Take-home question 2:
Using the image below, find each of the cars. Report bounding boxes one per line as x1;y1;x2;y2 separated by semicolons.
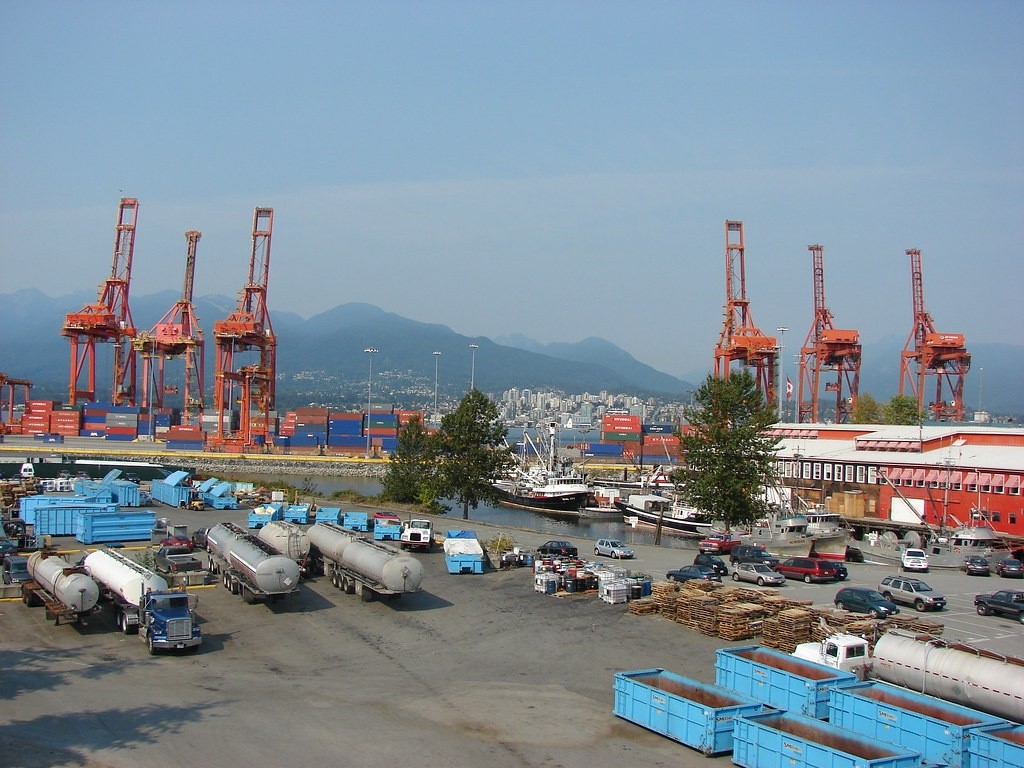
537;540;578;558
773;557;835;583
374;511;400;522
834;587;897;620
666;565;722;584
964;556;990;575
593;539;634;559
994;558;1024;578
160;536;194;554
731;562;786;586
830;561;848;581
694;554;728;576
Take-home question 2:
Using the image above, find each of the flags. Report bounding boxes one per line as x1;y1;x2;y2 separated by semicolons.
785;373;793;400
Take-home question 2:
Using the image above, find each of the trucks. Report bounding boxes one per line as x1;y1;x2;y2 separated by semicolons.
400;519;434;553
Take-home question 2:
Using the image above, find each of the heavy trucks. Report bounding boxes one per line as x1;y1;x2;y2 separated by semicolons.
787;626;1024;725
76;548;203;655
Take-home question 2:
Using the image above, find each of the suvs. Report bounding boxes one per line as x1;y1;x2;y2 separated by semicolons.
2;556;33;585
192;528;211;548
729;545;780;568
900;548;929;572
878;575;946;612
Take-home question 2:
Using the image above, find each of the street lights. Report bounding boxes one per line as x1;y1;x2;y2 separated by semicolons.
433;351;441;427
363;348;379;459
469;342;479;391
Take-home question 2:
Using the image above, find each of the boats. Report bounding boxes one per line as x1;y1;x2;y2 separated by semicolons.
480;421;1024;572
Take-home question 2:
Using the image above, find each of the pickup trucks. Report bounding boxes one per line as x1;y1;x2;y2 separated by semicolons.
973;590;1024;624
153;546;203;574
698;533;741;555
